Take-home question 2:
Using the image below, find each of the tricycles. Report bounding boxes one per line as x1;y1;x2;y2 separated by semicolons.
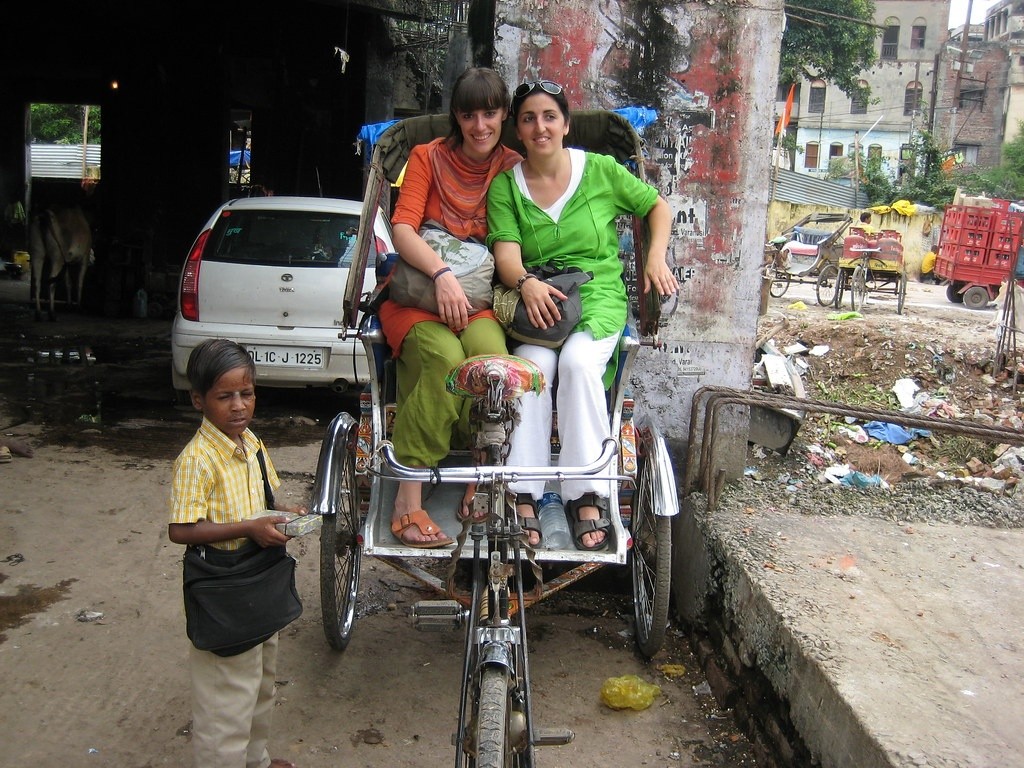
763;211;908;312
310;106;685;768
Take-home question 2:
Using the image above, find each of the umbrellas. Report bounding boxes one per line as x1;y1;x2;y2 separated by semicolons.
230;150;250;167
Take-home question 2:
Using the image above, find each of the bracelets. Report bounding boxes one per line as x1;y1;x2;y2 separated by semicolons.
432;267;452;283
515;273;537;293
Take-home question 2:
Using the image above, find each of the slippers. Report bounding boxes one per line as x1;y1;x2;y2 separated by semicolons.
391;509;454;549
457;490;488;524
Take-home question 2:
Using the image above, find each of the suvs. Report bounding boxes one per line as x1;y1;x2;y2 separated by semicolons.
170;195;399;415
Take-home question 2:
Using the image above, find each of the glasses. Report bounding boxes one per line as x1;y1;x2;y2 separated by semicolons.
513;80;566;98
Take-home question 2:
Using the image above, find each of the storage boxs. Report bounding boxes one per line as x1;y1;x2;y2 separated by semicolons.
940;185;1024;268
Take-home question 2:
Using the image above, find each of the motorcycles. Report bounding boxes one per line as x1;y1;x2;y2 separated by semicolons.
932;251;1024;310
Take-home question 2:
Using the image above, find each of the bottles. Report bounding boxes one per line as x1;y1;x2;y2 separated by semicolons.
537;491;570;550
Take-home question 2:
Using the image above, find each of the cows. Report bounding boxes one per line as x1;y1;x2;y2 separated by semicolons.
28;200;92;322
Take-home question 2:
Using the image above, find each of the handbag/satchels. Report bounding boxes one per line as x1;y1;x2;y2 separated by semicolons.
182;535;304;657
390;217;495;318
493;257;592;348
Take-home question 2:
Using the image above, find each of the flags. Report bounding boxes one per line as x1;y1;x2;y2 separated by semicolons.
774;84;796;135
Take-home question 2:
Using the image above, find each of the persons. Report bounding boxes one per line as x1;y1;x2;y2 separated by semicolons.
377;67;524;549
486;80;680;552
858;211;876;235
168;339;310;768
920;245;941;285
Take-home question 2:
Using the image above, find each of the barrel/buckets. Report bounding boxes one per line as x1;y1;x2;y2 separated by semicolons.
14;251;30;273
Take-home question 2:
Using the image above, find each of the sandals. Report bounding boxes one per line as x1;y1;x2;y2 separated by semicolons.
510;493;543;549
566;494;610;551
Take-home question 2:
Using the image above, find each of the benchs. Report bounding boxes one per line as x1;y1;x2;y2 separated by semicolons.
368;255;639;404
844;227;901;262
785;226;833;255
